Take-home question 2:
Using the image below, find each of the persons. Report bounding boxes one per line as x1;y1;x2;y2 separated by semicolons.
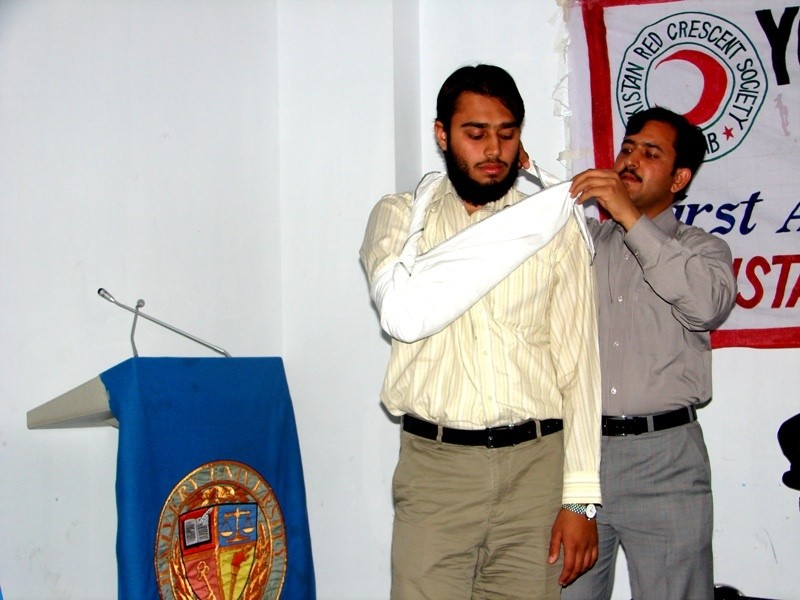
520;106;735;600
360;66;602;600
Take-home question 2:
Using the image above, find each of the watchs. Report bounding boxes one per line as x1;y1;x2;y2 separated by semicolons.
562;503;597;520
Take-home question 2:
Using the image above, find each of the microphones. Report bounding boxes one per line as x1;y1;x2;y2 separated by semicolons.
98;288;232;358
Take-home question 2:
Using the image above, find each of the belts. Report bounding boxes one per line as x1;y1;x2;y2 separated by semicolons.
403;414;563;448
602;404;697;436
